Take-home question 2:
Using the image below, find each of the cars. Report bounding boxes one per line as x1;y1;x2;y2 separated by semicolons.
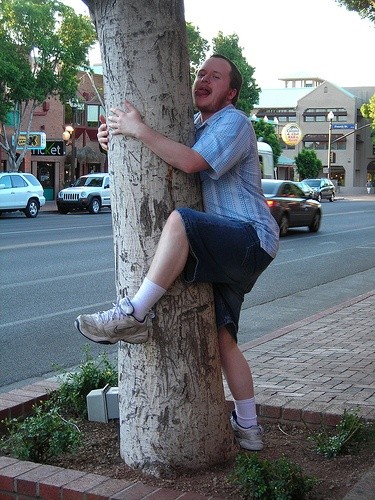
261;178;322;236
301;179;336;203
0;172;46;218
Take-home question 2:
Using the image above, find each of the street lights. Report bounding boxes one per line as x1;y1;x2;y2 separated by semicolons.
62;98;78;184
328;111;334;179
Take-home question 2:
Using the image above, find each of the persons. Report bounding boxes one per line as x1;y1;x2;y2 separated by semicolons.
75;53;280;449
329;174;371;194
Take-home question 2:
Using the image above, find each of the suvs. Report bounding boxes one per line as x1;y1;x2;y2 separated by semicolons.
56;173;111;214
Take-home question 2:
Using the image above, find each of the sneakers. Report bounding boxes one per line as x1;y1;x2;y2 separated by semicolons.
75;297;155;344
230;410;263;450
